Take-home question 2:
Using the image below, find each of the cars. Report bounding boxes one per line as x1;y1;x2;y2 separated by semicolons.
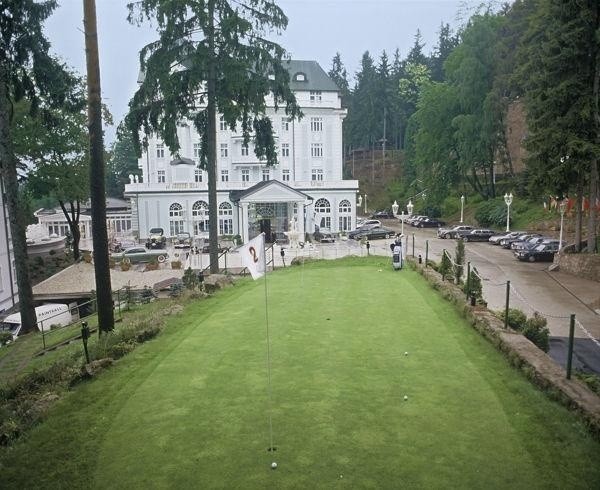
146;228;166;248
348;219;395;240
173;232;191;248
406;215;446;228
489;231;567;263
370;211;395;219
437;225;495;242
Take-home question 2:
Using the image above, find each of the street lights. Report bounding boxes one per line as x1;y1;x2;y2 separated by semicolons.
559;201;567;251
460;195;464;222
392;200;414;234
504;192;513;232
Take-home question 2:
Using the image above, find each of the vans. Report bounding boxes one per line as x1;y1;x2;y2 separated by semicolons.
0;304;71;344
